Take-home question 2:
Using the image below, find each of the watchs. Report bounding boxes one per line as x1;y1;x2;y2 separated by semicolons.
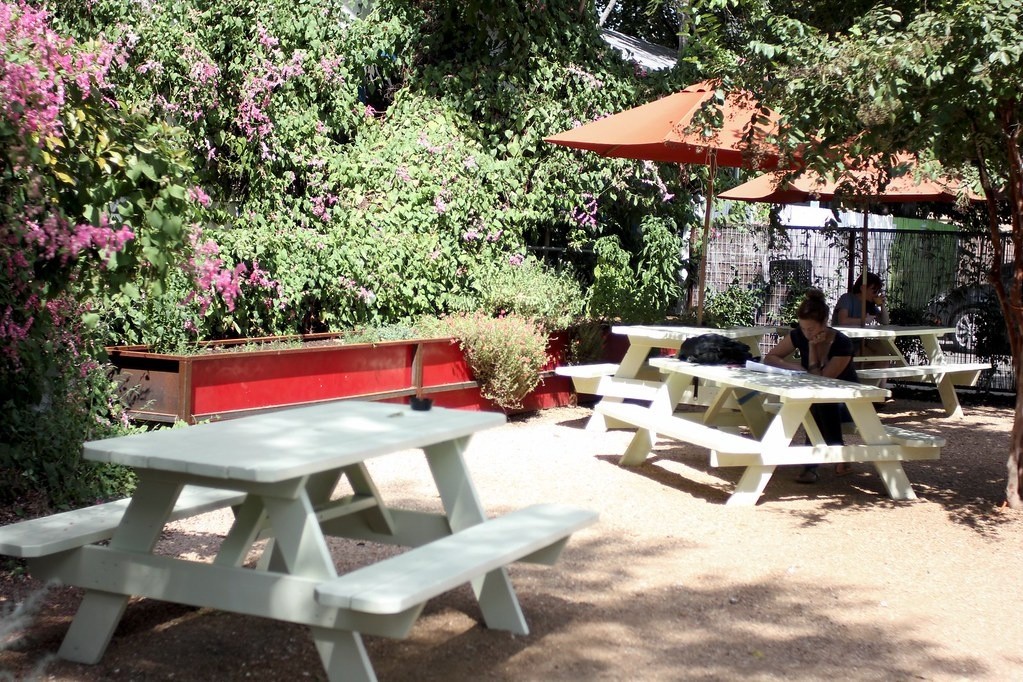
808;360;821;371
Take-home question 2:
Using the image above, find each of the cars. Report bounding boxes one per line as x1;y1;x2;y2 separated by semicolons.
922;284;999;354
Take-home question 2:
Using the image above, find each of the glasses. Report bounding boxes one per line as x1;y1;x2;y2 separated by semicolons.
869;287;881;294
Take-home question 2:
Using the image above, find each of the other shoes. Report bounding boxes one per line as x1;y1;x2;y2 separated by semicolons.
831;463;855;477
794;468;823;483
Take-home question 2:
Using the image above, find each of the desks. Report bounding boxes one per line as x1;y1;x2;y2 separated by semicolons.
55;400;530;682
619;358;918;509
585;326;779;438
777;325;982;417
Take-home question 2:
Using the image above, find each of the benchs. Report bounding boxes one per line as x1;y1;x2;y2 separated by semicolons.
852;356;901;363
763;404;945;448
556;363;621;379
314;503;599;613
856;362;992;381
595;402;762;453
0;483;248;558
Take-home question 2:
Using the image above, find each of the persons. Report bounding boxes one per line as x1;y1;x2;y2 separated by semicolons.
763;290;860;484
831;272;895;411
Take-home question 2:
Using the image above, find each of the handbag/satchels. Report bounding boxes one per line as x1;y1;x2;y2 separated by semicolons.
675;332;756;366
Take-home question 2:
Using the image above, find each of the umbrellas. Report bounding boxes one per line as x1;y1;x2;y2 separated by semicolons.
717;140;988;328
544;77;874;327
